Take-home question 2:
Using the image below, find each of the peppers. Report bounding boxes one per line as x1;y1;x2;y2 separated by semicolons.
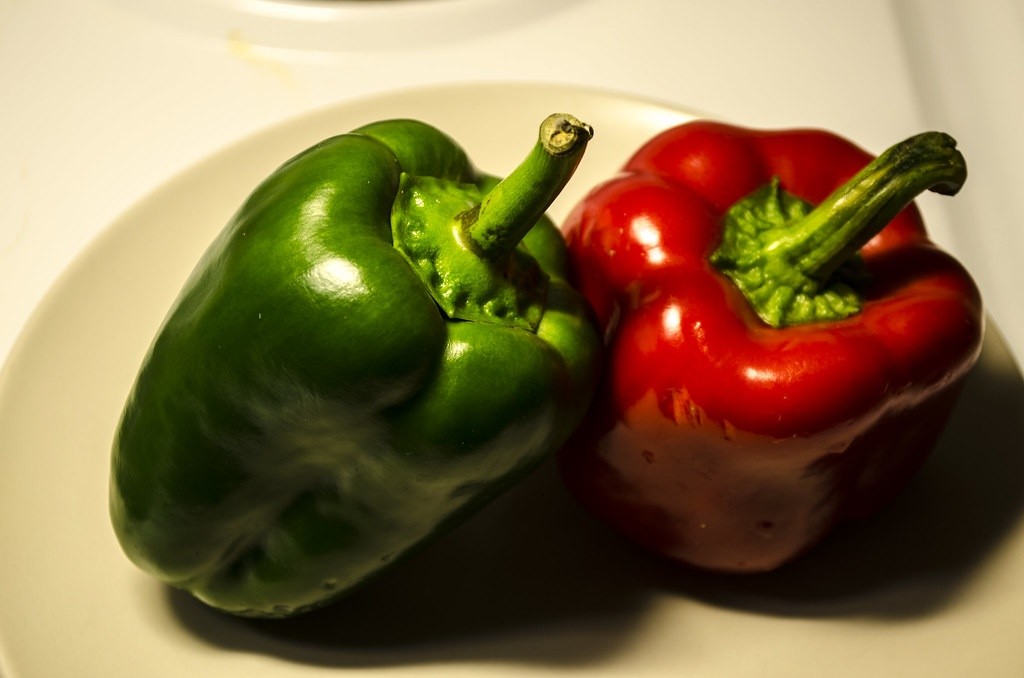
554;117;985;574
107;112;599;619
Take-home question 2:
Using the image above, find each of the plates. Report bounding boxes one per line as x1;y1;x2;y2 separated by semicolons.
0;81;1024;678
